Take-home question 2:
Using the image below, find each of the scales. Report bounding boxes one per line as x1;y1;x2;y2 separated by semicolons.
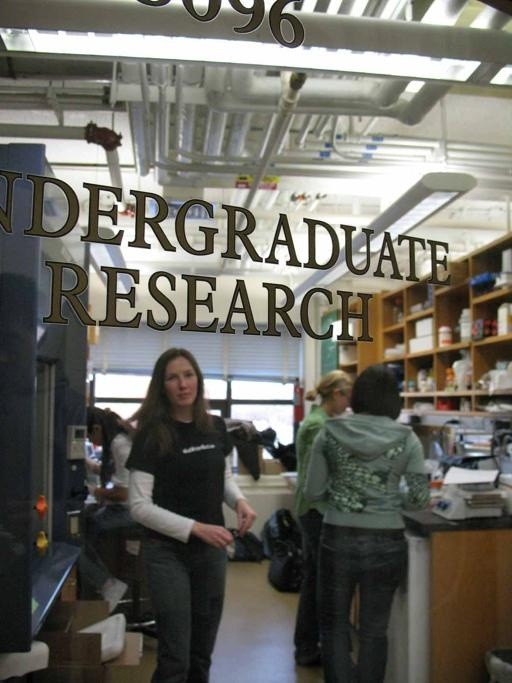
432;488;505;520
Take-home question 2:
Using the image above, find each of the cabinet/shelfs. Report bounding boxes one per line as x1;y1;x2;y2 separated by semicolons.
338;230;512;418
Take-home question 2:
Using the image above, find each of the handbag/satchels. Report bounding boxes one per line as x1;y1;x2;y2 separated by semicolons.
227;529;264;561
261;510;302;561
268;540;305;592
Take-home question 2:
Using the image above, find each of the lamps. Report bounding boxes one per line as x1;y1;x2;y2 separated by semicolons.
281;98;479;308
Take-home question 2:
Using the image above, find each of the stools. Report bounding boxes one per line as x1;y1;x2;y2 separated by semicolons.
80;503;158;632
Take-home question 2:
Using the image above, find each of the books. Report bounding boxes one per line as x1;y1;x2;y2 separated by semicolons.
443;466;507;509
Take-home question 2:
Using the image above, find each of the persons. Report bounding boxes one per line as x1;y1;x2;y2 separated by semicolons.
124;347;258;683
293;369;355;667
79;406;139;616
303;365;430;683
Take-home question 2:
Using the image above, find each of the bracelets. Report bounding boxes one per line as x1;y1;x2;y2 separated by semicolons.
93;463;97;474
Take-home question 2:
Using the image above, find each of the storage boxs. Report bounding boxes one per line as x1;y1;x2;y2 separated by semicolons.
34;600;160;683
59;565;77;604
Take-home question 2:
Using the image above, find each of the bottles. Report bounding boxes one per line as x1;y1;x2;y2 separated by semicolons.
438;325;452;346
417;367;427;392
457;309;471;344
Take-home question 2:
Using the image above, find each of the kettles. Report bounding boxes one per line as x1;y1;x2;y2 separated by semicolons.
441;419;465;458
